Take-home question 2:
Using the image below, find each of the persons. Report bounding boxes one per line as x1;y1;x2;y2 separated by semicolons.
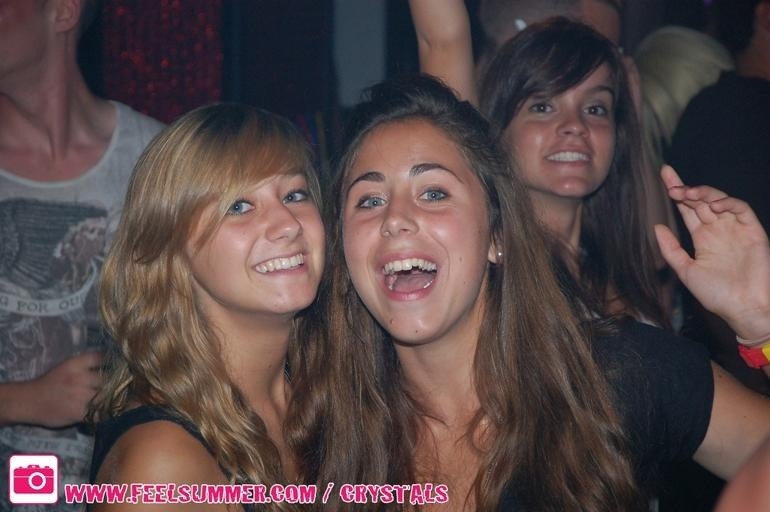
274;70;770;511
472;15;700;347
0;1;174;512
87;98;332;511
408;0;643;124
660;0;770;396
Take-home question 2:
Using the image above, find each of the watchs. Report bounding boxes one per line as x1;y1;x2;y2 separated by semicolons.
736;341;770;371
